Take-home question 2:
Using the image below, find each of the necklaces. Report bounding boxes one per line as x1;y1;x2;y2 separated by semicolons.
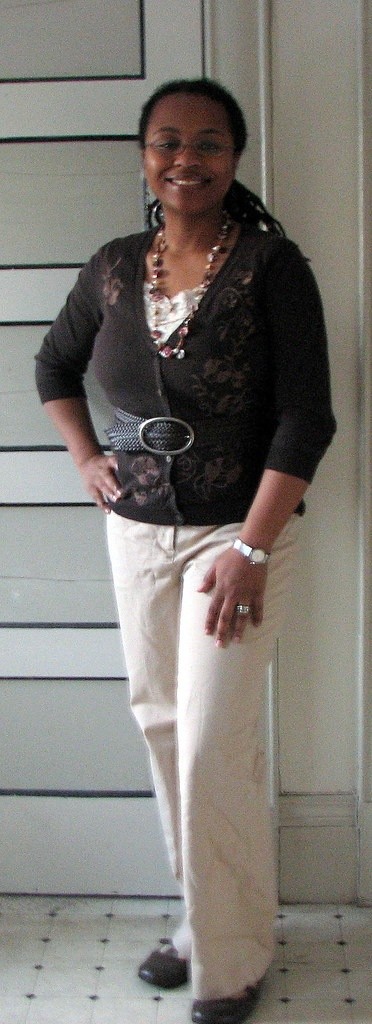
149;219;233;359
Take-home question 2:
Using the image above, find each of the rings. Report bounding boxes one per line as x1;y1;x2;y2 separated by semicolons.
237;605;251;613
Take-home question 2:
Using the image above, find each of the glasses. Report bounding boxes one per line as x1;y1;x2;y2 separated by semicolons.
143;137;237;158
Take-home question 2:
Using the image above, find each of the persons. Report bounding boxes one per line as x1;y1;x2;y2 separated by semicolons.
34;76;337;1024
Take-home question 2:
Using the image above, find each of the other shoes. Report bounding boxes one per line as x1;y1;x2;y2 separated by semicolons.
136;937;191;990
190;973;266;1024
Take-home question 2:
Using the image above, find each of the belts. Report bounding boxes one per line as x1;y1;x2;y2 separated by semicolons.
102;405;258;456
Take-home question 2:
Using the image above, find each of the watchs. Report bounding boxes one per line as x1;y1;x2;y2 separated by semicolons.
233;537;270;565
